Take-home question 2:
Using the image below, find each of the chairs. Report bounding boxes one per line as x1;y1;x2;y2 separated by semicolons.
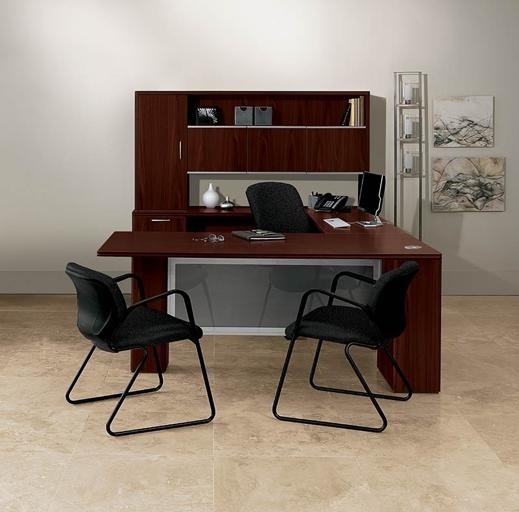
65;262;216;436
258;266;333;326
246;182;308;234
321;269;364;300
271;262;418;434
175;264;215;327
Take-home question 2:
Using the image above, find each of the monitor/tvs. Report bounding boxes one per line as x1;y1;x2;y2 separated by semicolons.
357;170;386;225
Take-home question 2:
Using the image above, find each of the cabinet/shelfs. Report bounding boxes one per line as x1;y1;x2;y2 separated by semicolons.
395;71;424;243
135;91;370;229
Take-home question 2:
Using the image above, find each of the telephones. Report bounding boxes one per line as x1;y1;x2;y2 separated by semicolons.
314;193;348;211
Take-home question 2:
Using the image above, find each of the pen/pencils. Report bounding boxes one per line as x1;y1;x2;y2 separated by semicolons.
311;191;318;196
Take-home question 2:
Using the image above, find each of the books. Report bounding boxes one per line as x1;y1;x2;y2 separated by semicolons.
231;229;287;241
338;94;364;128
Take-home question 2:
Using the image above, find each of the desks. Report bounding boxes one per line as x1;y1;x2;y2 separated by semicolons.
97;208;442;393
